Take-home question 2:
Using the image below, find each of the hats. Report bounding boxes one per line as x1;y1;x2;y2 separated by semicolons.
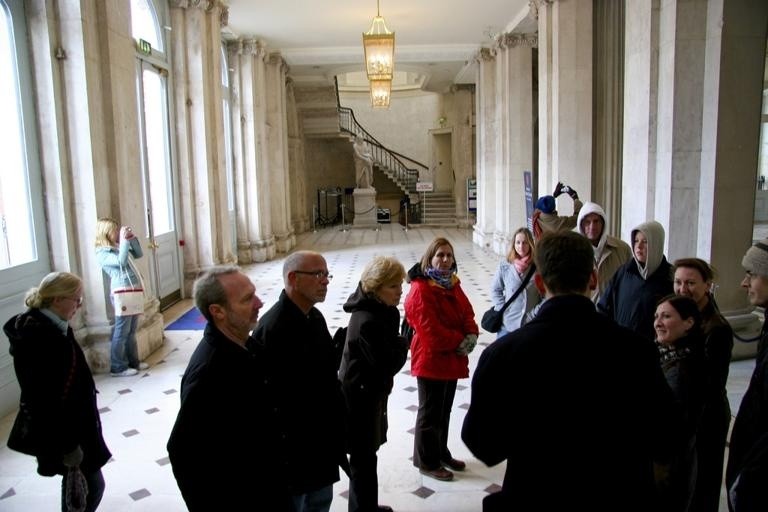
64;468;91;512
739;233;768;277
536;196;556;214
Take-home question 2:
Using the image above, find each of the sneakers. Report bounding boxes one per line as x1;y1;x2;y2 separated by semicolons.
137;360;150;370
109;367;139;377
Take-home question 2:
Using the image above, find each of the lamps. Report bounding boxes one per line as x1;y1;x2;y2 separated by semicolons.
369;80;393;110
357;0;395;80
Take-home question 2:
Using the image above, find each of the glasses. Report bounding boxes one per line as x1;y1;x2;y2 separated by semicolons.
292;270;334;280
56;295;84;305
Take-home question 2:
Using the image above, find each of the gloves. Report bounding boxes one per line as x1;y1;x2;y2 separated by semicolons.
567;186;578;199
553;182;565;197
62;447;86;469
455;334;476;357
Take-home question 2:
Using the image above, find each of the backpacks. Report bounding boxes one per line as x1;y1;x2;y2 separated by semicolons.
400;311;415;348
332;326;349;371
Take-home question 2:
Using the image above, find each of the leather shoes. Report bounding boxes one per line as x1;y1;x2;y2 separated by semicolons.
418;466;455;482
441;458;465;472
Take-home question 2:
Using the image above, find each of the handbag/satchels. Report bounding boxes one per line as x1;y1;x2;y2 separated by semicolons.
480;306;505;334
112;287;145;317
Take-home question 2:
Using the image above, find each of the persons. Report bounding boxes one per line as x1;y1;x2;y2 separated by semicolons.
725;238;768;511
353;132;375;189
3;271;112;511
252;251;345;511
653;295;713;511
577;201;632;312
339;255;413;510
534;181;584;232
492;227;548;340
95;216;150;375
461;230;695;511
599;219;676;345
673;258;734;511
405;237;478;481
166;264;309;510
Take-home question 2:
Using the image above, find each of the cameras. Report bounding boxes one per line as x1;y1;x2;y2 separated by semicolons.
125;226;134;233
561;186;570;193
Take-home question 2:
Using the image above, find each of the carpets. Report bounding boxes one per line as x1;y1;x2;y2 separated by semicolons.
166;299;211;333
